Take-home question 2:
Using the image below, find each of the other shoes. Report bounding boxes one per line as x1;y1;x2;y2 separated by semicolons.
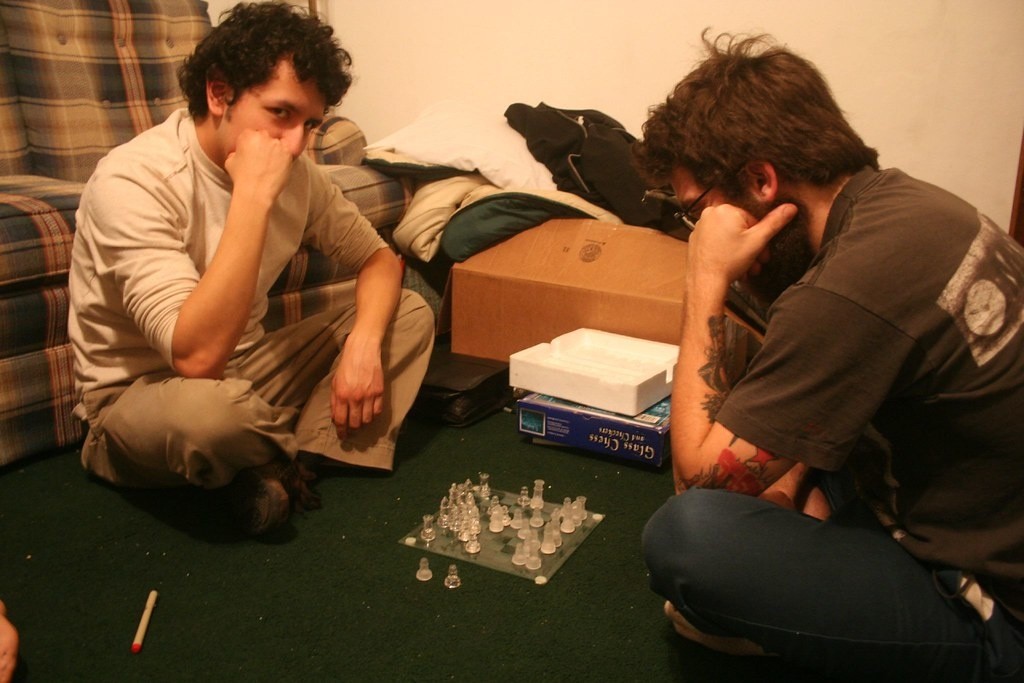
662;600;777;656
231;458;321;533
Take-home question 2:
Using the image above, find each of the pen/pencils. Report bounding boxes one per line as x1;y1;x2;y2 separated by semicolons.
131;586;161;651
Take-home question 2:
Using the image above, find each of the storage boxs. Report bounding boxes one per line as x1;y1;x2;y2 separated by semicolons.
516;393;672;470
435;218;689;362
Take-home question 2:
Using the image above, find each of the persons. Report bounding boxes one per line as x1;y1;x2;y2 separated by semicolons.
633;47;1024;683
67;0;437;534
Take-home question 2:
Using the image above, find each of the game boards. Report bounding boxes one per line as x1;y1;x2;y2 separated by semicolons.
396;485;608;585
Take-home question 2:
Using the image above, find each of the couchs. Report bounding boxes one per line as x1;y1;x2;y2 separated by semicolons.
0;0;435;465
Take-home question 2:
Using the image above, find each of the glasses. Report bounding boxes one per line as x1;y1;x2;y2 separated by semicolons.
673;181;720;231
